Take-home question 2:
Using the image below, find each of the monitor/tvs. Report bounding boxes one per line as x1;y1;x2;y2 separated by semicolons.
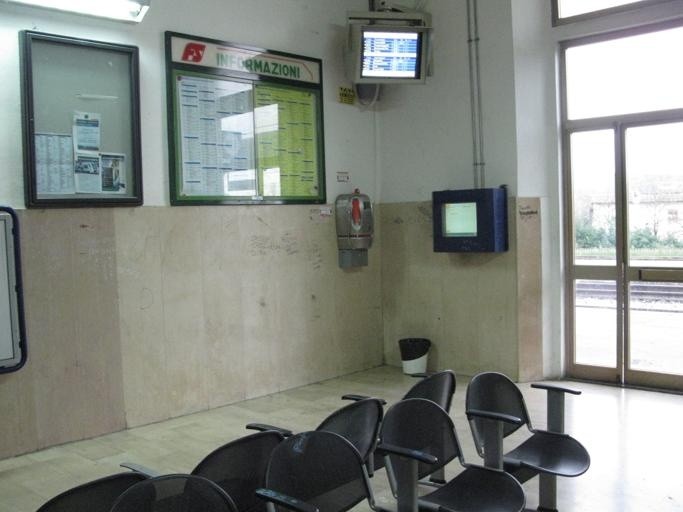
346;23;428;84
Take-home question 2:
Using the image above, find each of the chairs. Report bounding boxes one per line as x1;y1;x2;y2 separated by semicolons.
255;427;439;512
247;395;387;501
343;369;459;498
112;473;318;512
39;461;170;512
380;399;528;511
120;421;295;512
467;371;590;511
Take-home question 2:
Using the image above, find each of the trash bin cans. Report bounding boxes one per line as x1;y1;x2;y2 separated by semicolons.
399;338;431;374
